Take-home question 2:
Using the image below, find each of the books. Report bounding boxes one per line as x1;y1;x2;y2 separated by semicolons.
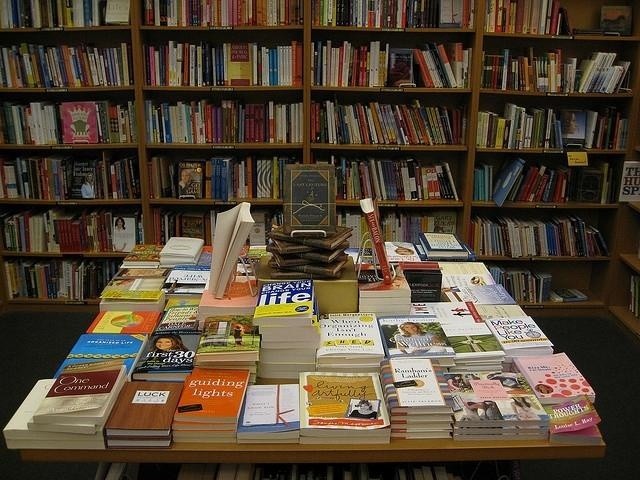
144;1;304;243
0;197;601;478
310;1;475;247
281;164;338;238
0;1;144;298
468;2;638;317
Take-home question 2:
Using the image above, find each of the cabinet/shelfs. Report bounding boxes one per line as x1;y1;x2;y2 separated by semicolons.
1;0;639;340
